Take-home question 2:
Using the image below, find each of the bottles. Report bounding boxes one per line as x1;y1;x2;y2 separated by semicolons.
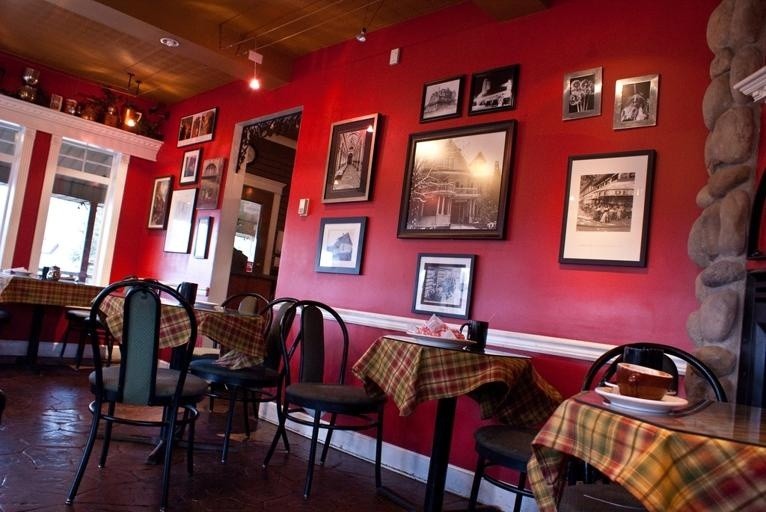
46;265;61;281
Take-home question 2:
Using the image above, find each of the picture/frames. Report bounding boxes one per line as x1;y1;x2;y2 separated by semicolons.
558;148;655;267
148;175;174;230
410;252;477;321
180;147;202;184
163;189;198;254
314;216;368;275
563;66;603;120
397;118;517;240
196;156;225;210
467;63;521;116
419;73;464;124
194;215;211;259
612;72;660;130
320;112;381;204
177;108;217;148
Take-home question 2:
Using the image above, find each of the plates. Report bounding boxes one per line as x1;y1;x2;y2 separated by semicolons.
194;301;218;310
407;332;477;352
595;387;688;414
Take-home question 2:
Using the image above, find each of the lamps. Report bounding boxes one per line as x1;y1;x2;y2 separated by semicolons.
354;33;366;43
161;37;180;48
124;106;142;127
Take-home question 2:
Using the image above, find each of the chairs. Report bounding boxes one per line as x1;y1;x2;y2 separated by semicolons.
64;278;209;512
59;277;157;368
574;341;727;512
259;300;385;501
169;292;273;428
472;341;629;512
188;296;304;465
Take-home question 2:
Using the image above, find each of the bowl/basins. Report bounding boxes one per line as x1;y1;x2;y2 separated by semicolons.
616;362;672;401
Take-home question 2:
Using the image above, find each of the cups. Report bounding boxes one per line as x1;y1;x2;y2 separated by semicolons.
175;281;198;308
459;320;489;352
622;347;665;374
